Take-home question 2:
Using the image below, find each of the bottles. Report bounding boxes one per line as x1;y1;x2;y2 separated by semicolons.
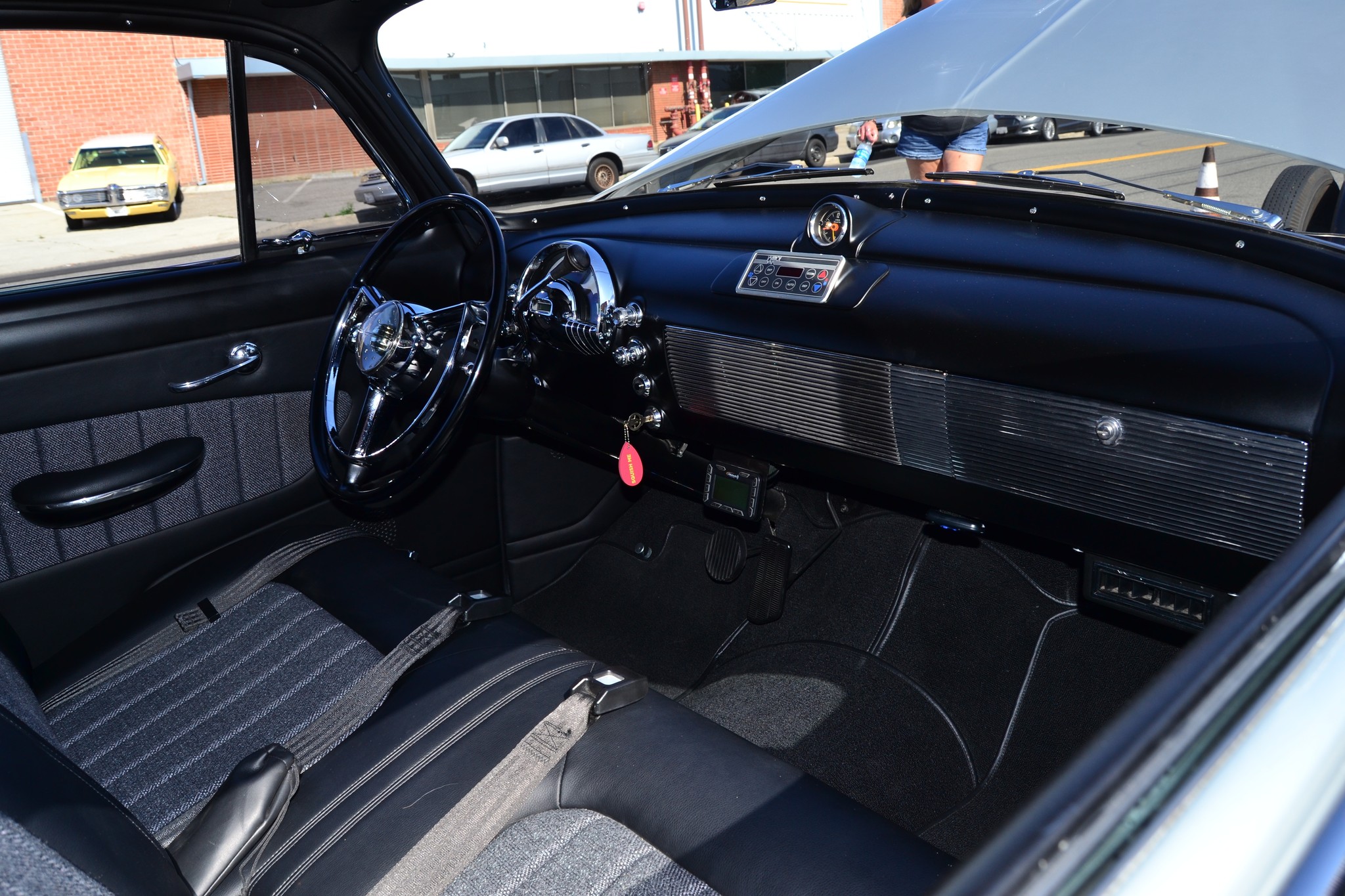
849;139;872;178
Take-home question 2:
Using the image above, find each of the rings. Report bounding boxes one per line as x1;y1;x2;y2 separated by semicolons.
865;129;870;132
872;134;878;137
857;134;861;137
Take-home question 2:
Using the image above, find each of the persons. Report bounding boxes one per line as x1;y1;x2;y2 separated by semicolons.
856;0;997;185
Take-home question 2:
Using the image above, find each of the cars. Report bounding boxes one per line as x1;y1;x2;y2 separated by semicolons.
993;115;1143;142
657;101;838;177
354;112;659;215
847;114;999;157
1;0;1345;895
57;133;184;230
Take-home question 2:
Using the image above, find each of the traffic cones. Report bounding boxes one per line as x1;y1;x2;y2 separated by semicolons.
1189;146;1222;217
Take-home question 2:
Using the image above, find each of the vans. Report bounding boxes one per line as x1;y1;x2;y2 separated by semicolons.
730;87;775;105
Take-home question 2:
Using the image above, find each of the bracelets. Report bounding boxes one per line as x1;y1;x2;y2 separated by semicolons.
860;119;876;126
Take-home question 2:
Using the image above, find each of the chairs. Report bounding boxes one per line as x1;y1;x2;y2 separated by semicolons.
0;493;970;896
